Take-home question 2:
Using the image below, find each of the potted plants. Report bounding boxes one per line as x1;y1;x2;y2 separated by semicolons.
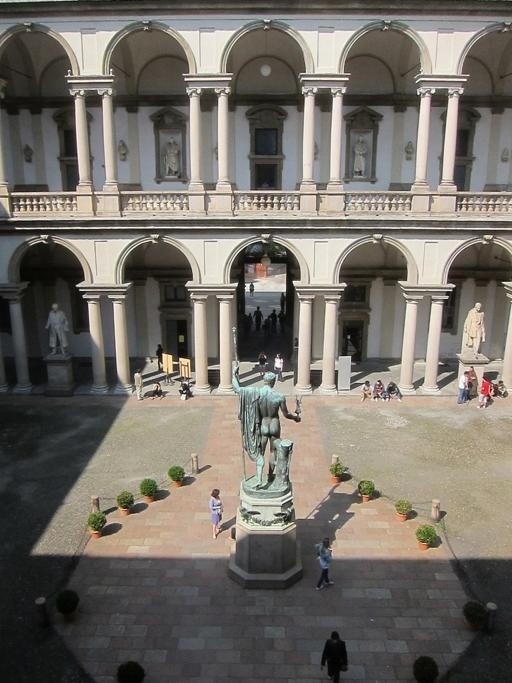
168;466;186;486
328;463;349;484
394;499;412;521
116;491;134;515
87;511;107;539
358;480;375;502
463;600;488;631
415;524;437;549
54;590;80;617
140;478;157;503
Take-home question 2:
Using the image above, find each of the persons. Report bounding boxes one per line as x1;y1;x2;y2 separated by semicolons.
178;384;193;398
258;351;267;380
386;382;401;401
243;306;285;336
164;137;180;176
45;303;69;356
464;303;485;359
209;489;224;539
232;361;301;490
280;292;286;312
315;538;334;590
352;135;368;176
152;382;163;398
321;631;348;683
457;365;506;408
250;282;254;297
373;380;386;402
156;344;163;371
360;381;375;402
134;369;143;400
274;354;284;383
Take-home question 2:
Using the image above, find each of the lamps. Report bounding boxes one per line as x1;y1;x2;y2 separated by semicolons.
260;29;272;76
